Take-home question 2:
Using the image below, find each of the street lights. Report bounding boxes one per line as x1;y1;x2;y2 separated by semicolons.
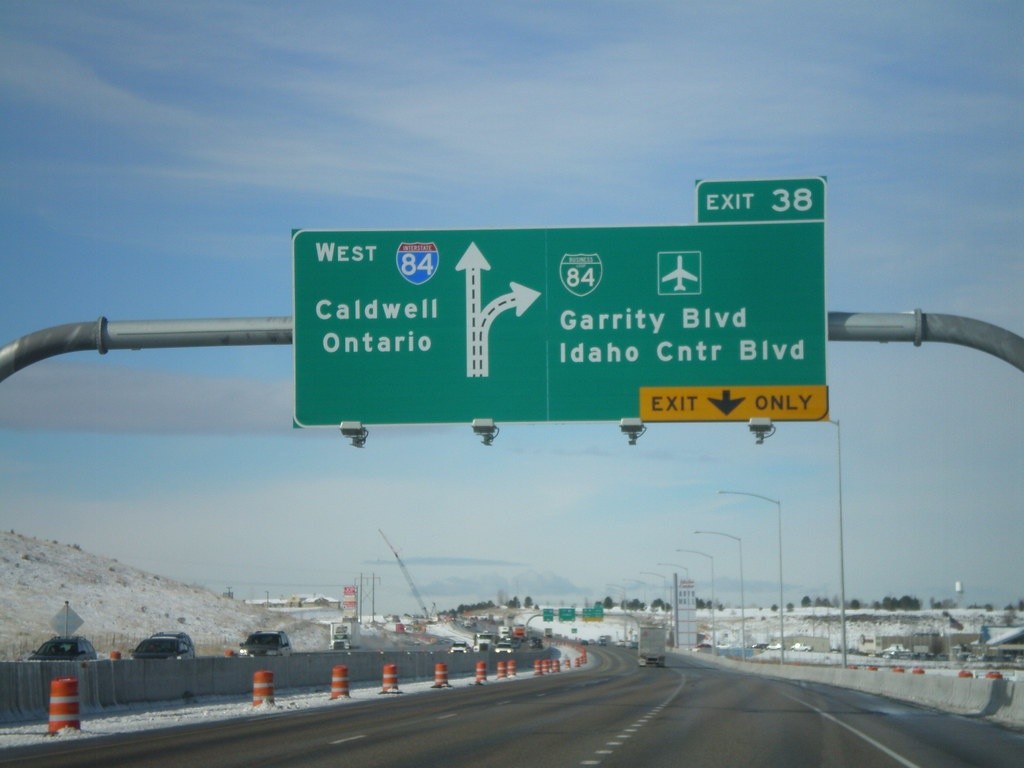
674;488;785;663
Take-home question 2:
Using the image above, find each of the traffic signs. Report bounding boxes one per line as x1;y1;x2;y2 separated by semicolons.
291;220;829;426
543;608;554;621
558;608;576;621
583;608;605;621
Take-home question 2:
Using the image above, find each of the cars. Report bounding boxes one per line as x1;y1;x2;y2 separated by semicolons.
494;642;516;653
863;647;1018;663
691;643;712;653
752;640;815;652
448;641;474;654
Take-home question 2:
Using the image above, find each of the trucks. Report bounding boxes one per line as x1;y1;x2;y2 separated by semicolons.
473;631;494;648
497;624;526;640
598;636;607;647
329;622;362;651
637;624;666;667
544;628;553;638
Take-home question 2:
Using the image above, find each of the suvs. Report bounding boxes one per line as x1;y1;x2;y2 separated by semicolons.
27;635;97;663
237;630;292;658
128;631;197;661
